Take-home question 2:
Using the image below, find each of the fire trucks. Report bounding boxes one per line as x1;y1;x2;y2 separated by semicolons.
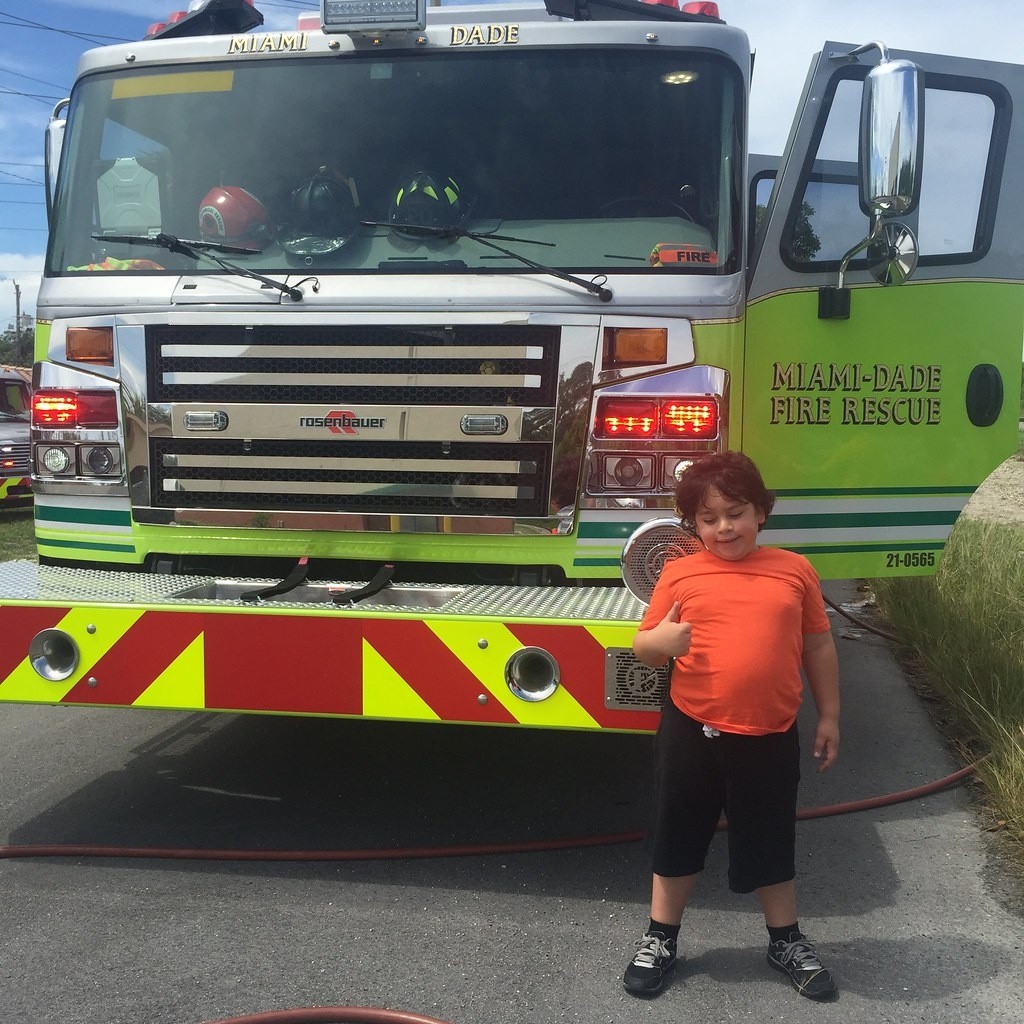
0;0;1024;735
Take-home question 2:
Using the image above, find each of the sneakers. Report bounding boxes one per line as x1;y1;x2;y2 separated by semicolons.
765;930;838;1002
621;930;678;998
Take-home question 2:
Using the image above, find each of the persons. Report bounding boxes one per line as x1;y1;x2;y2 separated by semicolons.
622;452;839;999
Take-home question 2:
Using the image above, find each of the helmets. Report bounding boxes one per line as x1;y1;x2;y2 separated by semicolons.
273;174;360;257
197;186;272;252
390;169;473;242
